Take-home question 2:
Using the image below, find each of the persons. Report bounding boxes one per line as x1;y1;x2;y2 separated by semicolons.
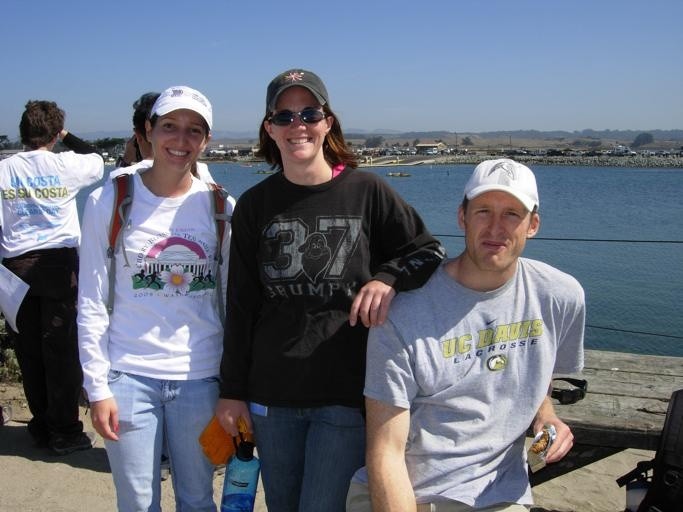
75;86;236;512
344;159;586;512
215;69;447;512
0;101;104;458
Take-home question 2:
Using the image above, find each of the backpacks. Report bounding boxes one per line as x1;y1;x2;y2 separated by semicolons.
615;389;682;512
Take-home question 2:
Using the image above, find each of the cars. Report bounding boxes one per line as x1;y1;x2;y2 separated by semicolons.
379;143;417;155
514;149;527;154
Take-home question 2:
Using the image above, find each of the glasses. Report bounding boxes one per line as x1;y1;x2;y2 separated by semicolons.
551;376;588;406
271;107;325;126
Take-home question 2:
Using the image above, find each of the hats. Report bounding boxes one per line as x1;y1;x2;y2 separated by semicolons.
463;158;539;213
266;69;329;112
149;84;214;144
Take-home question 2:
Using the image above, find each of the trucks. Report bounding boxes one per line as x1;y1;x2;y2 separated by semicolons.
567;146;683;157
426;144;468;155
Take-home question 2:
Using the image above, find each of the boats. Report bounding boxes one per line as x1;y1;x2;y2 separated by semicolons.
248;171;273;175
385;172;411;177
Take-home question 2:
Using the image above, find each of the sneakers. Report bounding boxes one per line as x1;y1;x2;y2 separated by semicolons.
49;430;98;456
2;406;12;422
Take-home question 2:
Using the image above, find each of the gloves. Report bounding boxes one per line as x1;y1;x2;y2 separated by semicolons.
199;413;251;465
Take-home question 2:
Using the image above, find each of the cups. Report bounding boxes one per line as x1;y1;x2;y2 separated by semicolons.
220;430;260;512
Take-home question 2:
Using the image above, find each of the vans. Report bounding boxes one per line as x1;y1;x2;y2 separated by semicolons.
546;148;565;156
